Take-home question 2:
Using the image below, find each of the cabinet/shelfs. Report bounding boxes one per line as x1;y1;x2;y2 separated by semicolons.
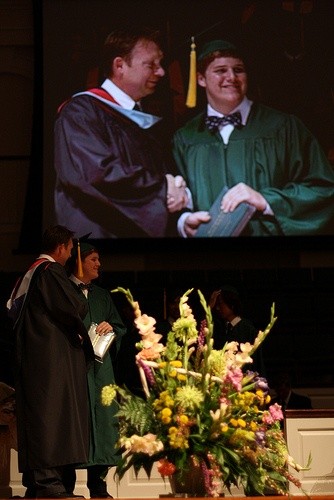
8;409;334;499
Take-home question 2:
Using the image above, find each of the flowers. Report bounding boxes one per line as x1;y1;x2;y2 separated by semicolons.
96;286;314;500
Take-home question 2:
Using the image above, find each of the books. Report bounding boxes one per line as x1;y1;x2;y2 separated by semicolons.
192;182;257;233
82;321;116;364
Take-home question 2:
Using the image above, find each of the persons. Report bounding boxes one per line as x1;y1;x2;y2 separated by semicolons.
151;287;271;416
7;225;126;499
53;27;333;235
